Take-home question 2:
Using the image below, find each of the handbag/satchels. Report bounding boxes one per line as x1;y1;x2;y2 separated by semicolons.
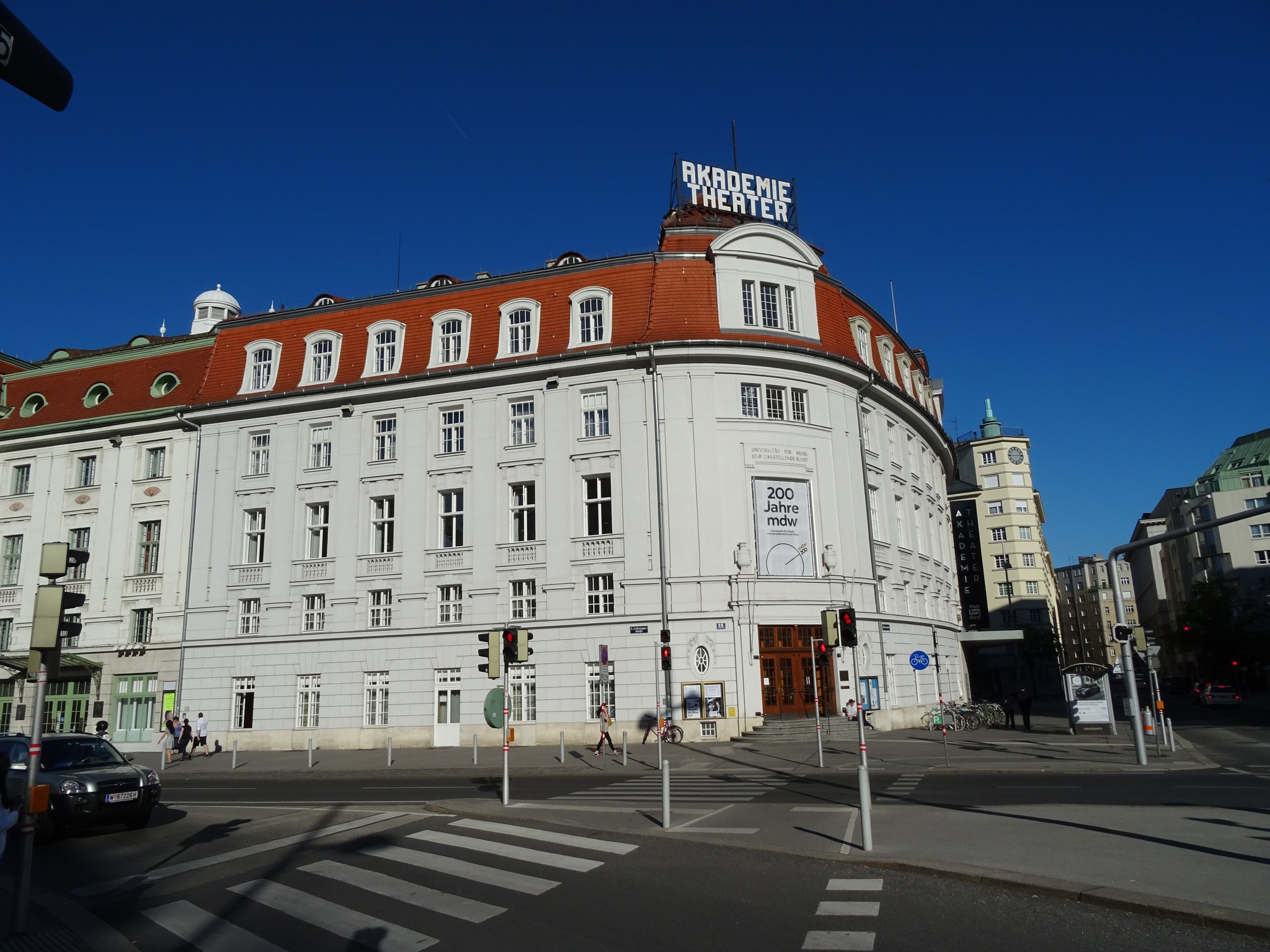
189;735;193;742
608;717;612;726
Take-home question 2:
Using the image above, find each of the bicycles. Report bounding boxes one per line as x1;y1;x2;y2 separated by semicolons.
642;714;684;745
921;697;1006;731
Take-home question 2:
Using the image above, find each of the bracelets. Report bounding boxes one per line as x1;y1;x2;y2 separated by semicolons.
197;736;200;738
178;739;180;741
159;740;160;742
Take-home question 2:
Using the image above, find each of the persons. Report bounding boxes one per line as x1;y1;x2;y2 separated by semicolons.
0;750;24;860
94;720;112;759
1001;686;1034;729
158;712;209;765
708;701;722;718
595;702;621;755
846;699;874;729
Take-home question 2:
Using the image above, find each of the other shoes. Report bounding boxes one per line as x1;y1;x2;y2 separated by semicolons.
872;727;879;730
594;753;602;756
173;752;178;754
179;758;185;761
165;761;172;764
203;754;210;759
614;751;621;756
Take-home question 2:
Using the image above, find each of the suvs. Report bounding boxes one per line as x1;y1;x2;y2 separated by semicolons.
1075;682;1100;698
0;730;162;843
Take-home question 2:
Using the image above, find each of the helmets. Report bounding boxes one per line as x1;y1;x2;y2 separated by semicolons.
96;721;108;732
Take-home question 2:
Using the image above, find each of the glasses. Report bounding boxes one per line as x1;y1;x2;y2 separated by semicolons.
605;706;608;708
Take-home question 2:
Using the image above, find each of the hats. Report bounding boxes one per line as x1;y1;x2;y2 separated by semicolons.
166;720;173;726
849;699;855;703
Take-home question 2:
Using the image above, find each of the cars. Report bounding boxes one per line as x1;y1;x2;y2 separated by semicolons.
1134;673;1195;695
1196;684;1240;709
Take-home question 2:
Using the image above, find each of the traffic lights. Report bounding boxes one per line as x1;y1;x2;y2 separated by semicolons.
838;608;858;648
1182;622;1189;634
30;585;86;651
478;629;500;680
517;629;533;664
661;646;671;670
39;541;90;580
502;627;518;663
1112;623;1133;643
817;639;831;667
